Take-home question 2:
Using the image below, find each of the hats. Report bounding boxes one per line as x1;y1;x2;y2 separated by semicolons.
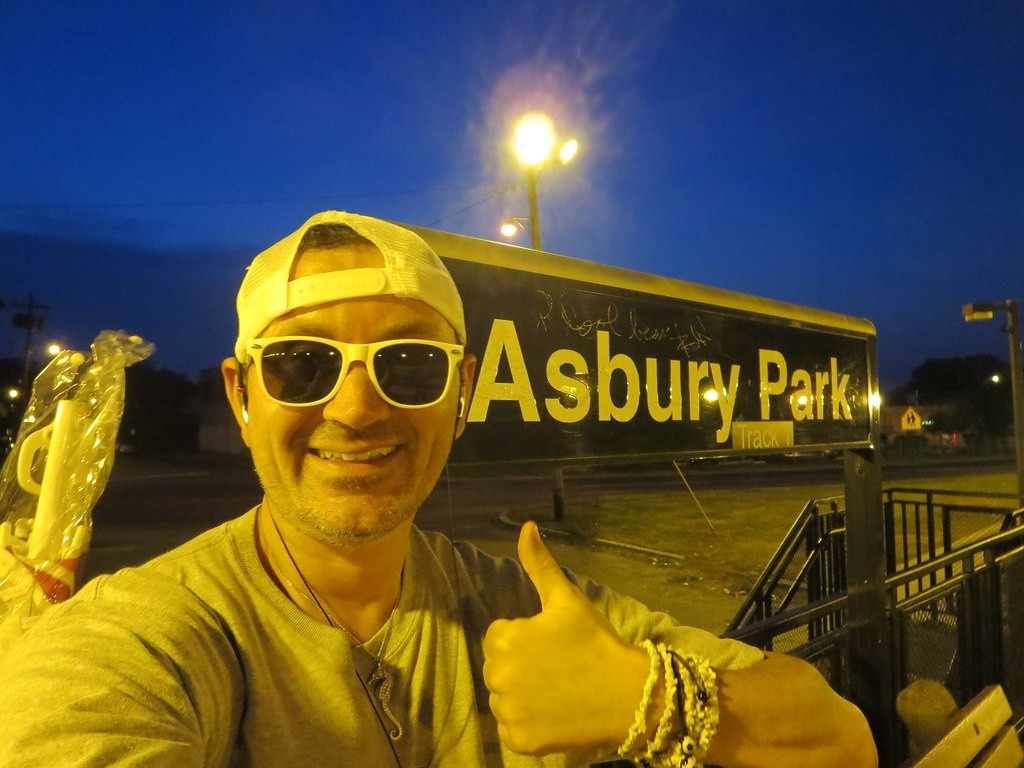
234;210;467;370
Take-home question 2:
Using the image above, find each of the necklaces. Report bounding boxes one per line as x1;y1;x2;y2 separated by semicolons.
287;576;402;740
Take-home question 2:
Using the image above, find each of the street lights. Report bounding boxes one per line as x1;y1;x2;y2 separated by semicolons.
960;297;1023;513
506;113;580;525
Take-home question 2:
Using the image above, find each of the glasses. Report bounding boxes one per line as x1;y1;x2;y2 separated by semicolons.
245;335;464;409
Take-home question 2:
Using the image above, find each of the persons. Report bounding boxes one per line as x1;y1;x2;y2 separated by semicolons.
0;207;882;768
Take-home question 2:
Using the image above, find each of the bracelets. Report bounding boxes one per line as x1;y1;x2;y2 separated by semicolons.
617;637;720;768
